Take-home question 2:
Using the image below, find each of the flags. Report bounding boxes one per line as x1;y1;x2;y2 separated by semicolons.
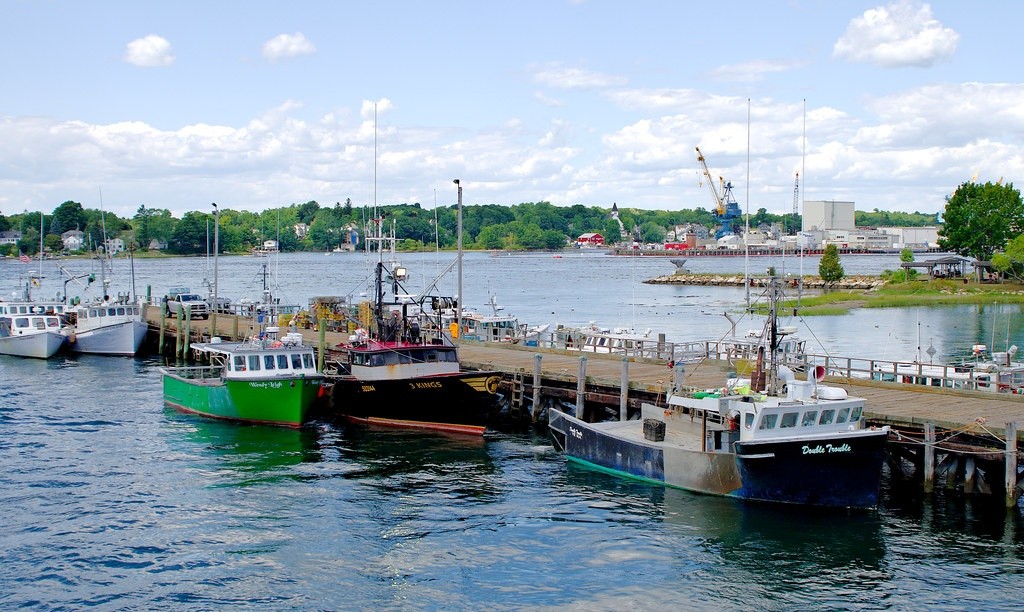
20;252;31;262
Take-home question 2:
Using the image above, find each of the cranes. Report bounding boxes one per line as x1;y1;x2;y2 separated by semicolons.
791;171;800;236
695;145;745;242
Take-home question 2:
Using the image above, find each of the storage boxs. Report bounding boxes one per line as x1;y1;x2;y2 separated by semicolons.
643;419;666;442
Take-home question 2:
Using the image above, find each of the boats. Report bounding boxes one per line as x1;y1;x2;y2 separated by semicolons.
548;97;893;509
0;101;653;440
718;327;808;373
870;299;1024;395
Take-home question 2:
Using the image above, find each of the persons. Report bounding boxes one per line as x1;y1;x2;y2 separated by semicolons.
162;295;170;303
989;272;997;284
311;304;318;331
